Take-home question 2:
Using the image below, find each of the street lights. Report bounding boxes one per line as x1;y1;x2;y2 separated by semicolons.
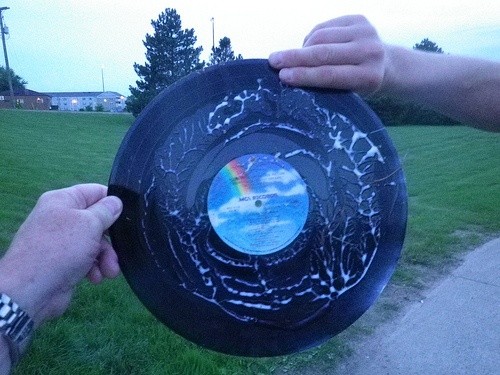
210;16;215;51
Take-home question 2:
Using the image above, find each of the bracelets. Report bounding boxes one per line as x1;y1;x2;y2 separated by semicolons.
0;291;35;365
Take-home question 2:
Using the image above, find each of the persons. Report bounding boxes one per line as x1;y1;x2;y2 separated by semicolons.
0;15;500;375
